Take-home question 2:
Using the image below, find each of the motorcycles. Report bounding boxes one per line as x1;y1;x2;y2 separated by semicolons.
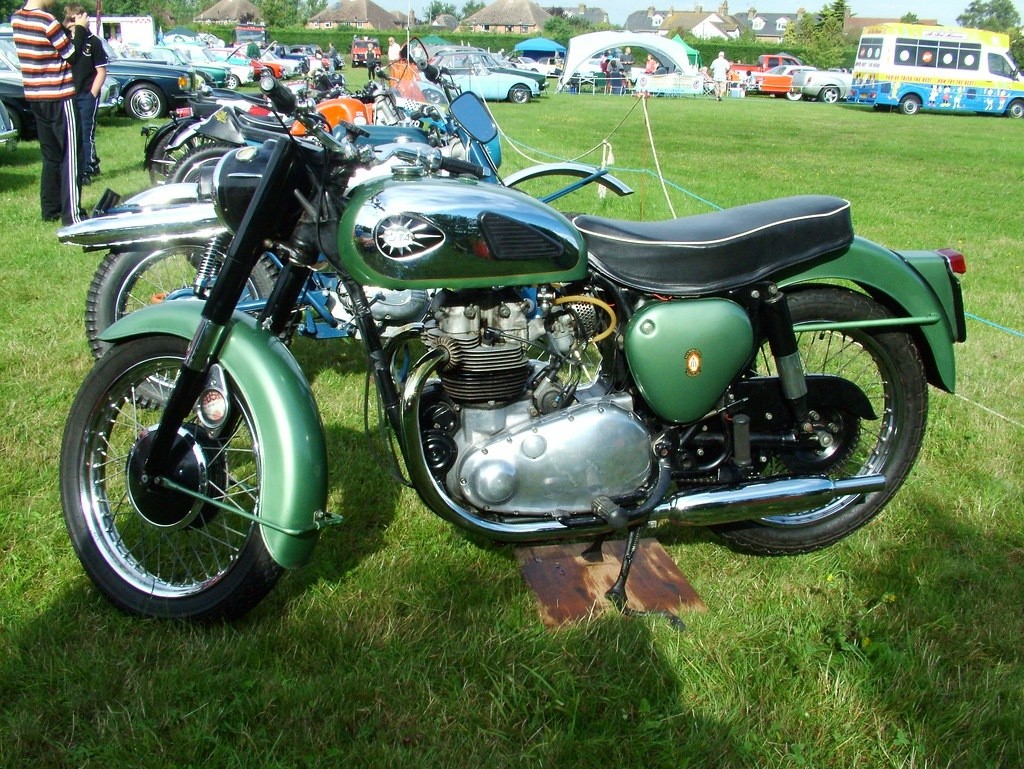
58;71;967;634
56;32;633;418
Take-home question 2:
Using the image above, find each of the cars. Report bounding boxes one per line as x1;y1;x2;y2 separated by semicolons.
0;13;548;158
751;63;817;100
790;65;853;105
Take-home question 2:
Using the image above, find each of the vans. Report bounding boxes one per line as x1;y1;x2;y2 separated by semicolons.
845;21;1024;117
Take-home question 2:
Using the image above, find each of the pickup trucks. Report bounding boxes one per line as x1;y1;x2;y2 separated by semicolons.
728;52;805;93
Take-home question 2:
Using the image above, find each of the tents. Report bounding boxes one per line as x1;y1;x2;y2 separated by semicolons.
514;38;567;63
670;33;703;68
554;29;691;95
413;32;453;46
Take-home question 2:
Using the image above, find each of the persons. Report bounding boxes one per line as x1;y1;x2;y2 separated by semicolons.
601;46;658;95
699;66;757;96
246;37;261;60
314;42;323;59
11;0;108;228
388;35;401;86
709;50;731;101
326;41;336;71
365;42;376;82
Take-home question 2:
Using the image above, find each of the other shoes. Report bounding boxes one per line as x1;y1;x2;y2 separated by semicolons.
81;175;92;186
91;167;101;176
717;95;722;101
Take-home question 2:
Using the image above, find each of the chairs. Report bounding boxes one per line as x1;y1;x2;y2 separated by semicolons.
570;73;581;94
595;72;627;95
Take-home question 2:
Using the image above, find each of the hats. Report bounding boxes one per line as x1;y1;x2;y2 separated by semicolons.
719;51;725;56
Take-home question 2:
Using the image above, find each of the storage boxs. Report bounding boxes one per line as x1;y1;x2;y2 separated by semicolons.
730;88;746;98
